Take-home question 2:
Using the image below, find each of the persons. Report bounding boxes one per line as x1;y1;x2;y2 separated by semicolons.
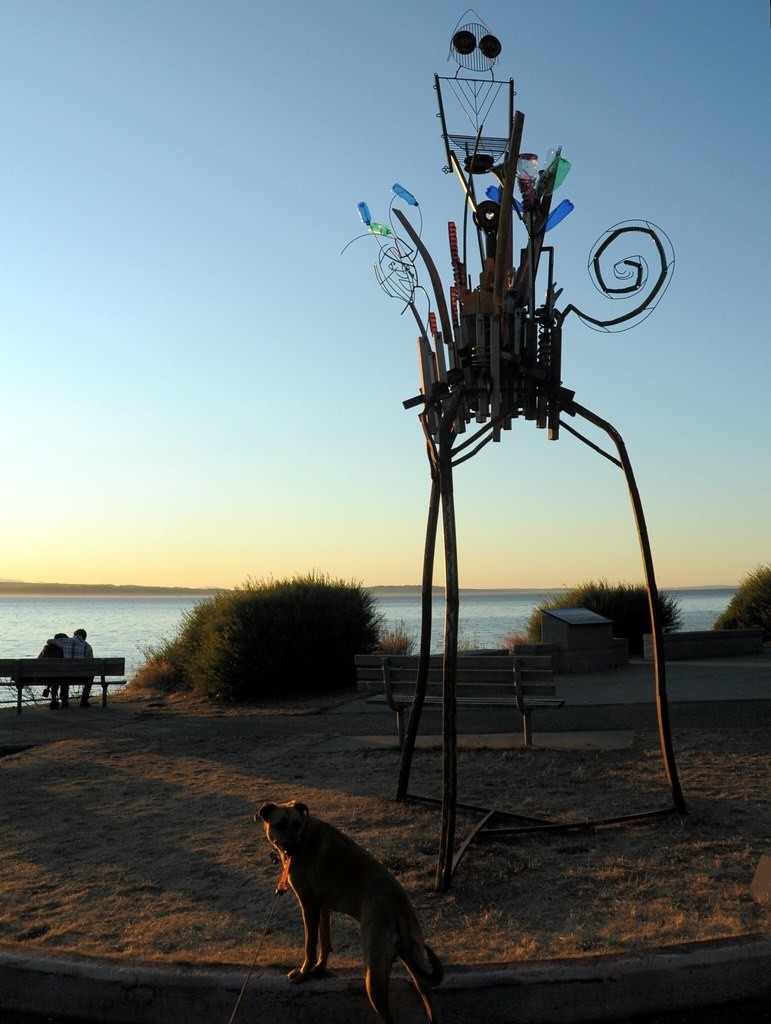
37;632;70;709
47;629;95;708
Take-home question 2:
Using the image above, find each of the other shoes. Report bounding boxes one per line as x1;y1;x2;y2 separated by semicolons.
43;688;93;709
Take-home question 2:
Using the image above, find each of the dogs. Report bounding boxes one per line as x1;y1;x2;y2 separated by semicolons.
254;801;443;1024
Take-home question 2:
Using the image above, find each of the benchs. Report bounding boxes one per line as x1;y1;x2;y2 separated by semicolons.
355;654;566;748
8;658;126;715
